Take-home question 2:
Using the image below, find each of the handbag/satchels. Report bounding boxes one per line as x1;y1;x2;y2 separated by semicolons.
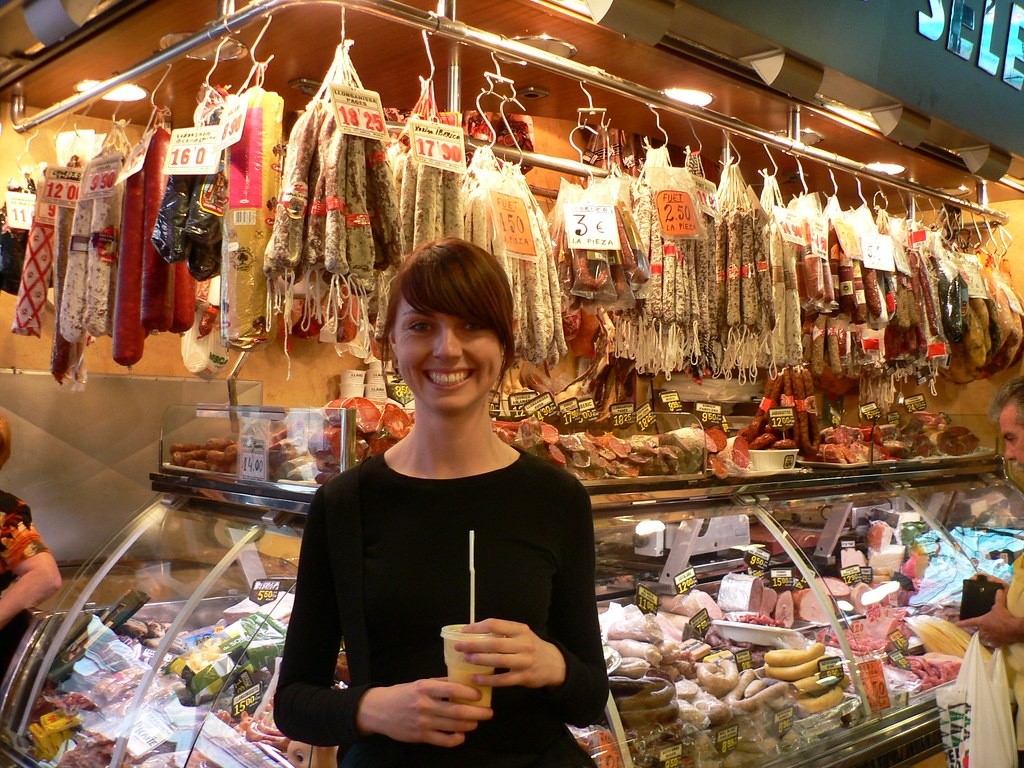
935;629;1019;768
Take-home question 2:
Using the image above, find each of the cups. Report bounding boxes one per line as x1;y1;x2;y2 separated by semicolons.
440;624;505;709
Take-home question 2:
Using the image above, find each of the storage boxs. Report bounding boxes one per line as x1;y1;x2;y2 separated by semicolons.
748;449;799;472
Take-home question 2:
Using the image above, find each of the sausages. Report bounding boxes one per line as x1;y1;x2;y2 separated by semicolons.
597;621;852;735
50;103;968;385
169;366;980;483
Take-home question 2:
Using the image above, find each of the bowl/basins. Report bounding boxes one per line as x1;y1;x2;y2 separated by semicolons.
750;449;799;471
339;361;387;402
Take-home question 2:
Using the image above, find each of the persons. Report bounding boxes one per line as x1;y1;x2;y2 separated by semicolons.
0;406;63;688
272;234;610;768
954;373;1024;768
